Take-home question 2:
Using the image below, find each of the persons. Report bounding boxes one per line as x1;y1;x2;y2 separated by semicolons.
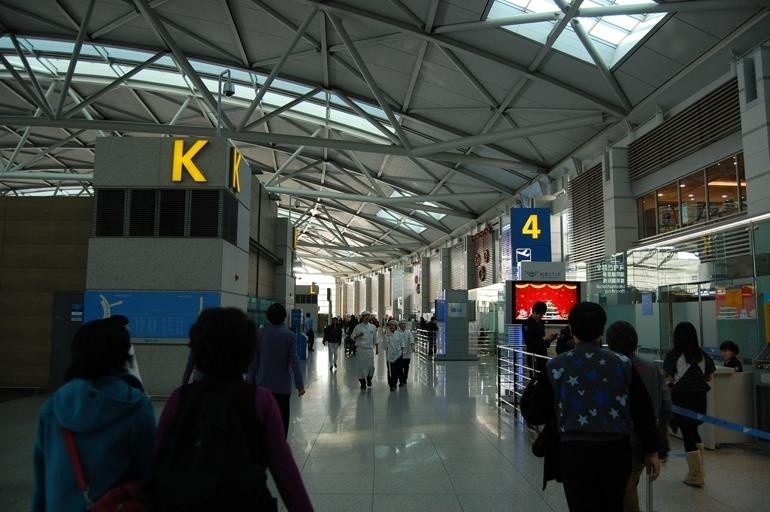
478;328;485;344
109;314;144;387
28;319;154;512
322;311;438;392
720;340;743;372
520;302;717;510
246;303;305;441
154;306;315;512
305;313;315;351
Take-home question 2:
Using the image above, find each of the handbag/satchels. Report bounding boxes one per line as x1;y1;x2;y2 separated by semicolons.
87;482;145;512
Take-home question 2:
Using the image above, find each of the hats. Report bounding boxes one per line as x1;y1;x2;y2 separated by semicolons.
362;311;370;315
389;319;406;325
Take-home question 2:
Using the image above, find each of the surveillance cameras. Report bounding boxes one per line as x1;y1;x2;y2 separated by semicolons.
294;201;300;209
222;81;236;98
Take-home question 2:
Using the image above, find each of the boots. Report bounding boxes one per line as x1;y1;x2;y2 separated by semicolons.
683;443;705;487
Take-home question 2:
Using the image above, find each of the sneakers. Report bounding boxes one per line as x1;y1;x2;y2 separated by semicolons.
368;380;371;385
361;383;366;390
390;380;406;391
